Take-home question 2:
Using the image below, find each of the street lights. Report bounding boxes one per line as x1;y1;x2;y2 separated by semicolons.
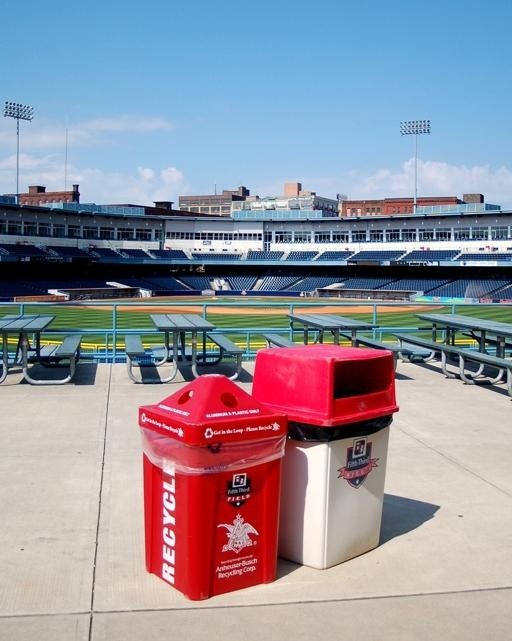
3;100;35;194
400;119;432;214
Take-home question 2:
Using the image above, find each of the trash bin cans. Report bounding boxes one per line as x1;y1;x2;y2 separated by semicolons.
250;344;399;570
140;375;289;601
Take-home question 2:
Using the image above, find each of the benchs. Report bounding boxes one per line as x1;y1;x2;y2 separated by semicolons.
56;330;511;372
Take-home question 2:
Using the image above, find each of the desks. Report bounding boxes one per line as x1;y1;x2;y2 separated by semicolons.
0;314;81;385
124;313;242;384
413;314;511;396
287;314;397;375
